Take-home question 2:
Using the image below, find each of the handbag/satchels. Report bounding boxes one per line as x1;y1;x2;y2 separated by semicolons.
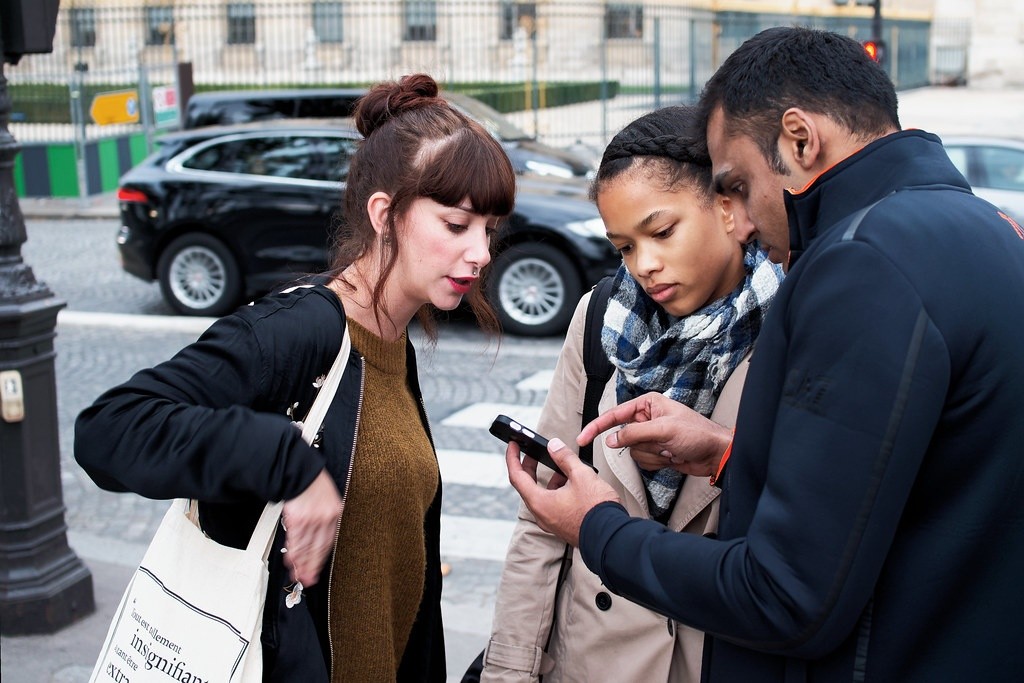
88;283;352;683
460;648;543;683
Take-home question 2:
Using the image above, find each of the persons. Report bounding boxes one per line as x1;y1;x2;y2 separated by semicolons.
505;28;1024;683
480;109;788;683
72;73;516;683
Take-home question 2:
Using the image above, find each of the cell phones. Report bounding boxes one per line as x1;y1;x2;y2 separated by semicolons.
489;415;599;477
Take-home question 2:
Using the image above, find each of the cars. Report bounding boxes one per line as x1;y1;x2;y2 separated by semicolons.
114;117;625;340
938;135;1024;229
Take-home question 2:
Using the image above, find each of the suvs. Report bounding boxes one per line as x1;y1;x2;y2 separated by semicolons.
182;85;597;182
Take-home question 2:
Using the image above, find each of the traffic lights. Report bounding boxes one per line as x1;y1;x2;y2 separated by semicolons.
863;38;884;61
